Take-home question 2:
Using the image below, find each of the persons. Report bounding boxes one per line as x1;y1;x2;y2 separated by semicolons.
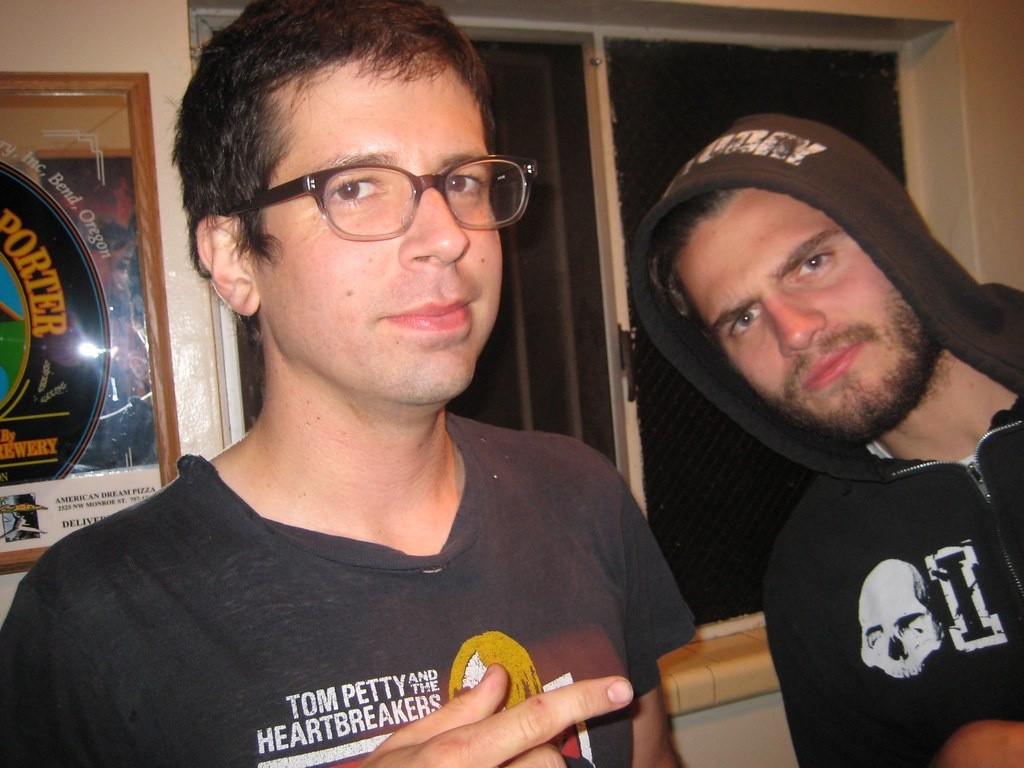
626;112;1024;768
0;0;700;768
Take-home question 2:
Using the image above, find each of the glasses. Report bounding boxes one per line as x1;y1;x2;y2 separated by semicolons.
221;155;538;242
